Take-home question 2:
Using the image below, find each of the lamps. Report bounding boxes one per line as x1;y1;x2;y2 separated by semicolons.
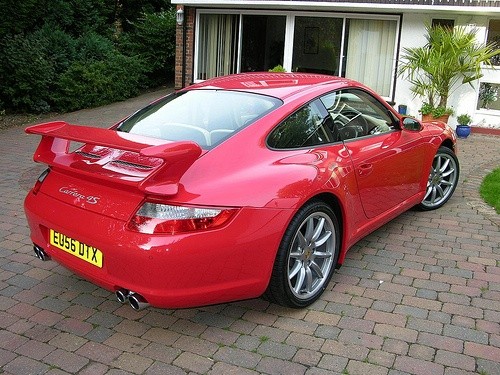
176;7;186;26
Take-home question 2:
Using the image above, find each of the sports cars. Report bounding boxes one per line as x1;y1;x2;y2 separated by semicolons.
22;70;460;312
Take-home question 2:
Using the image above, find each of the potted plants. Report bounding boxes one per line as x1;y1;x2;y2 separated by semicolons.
391;22;500;124
455;115;472;139
